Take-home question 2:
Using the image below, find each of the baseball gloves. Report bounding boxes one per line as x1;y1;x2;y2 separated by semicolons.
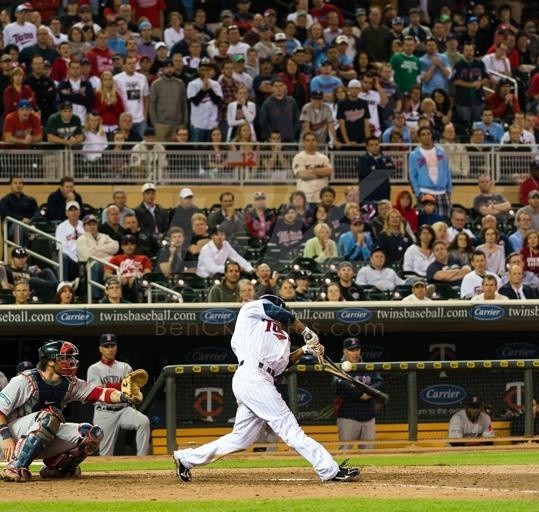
121;369;148;405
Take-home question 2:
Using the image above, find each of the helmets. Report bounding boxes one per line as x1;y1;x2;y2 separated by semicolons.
38;338;80;385
295;270;314;281
11;247;28;258
259;293;286;308
469;396;480;404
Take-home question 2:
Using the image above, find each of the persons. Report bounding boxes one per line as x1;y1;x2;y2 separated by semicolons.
0;127;539;301
172;294;360;482
88;332;150;456
511;397;539;445
0;0;538;181
335;337;385;448
0;340;140;483
444;394;495;446
16;361;34;376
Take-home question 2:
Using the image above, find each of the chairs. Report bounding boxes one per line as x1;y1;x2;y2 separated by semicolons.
1;198;538;306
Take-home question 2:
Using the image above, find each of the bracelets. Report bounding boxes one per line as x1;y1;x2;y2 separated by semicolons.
294;321;302;330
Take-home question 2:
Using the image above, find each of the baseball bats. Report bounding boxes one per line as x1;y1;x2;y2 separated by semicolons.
320;352;387;400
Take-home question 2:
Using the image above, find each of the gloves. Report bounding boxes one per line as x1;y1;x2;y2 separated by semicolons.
301;327;319;345
301;343;325;356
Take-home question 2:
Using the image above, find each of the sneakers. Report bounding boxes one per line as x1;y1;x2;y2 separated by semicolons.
0;467;21;482
40;466;81;478
173;450;192;482
329;465;360;482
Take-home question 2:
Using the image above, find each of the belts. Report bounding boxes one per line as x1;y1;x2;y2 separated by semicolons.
239;359;275;377
97;406;124;411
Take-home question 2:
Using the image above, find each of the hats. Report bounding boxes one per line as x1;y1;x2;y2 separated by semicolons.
14;4;28;13
311;34;361;101
155;41;167;52
17;99;30;108
17;361;33;372
420;194;435;204
25;3;34;11
60;101;73;110
221;9;287;63
355;4;480;25
180;188;195;200
528;189;539;199
142;183;157;192
338;215;426;287
0;54;13;62
254;191;266;200
56;201;137;293
100;333;118;346
344;338;361;348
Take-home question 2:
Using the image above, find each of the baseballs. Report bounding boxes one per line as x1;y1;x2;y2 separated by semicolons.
342;361;352;371
